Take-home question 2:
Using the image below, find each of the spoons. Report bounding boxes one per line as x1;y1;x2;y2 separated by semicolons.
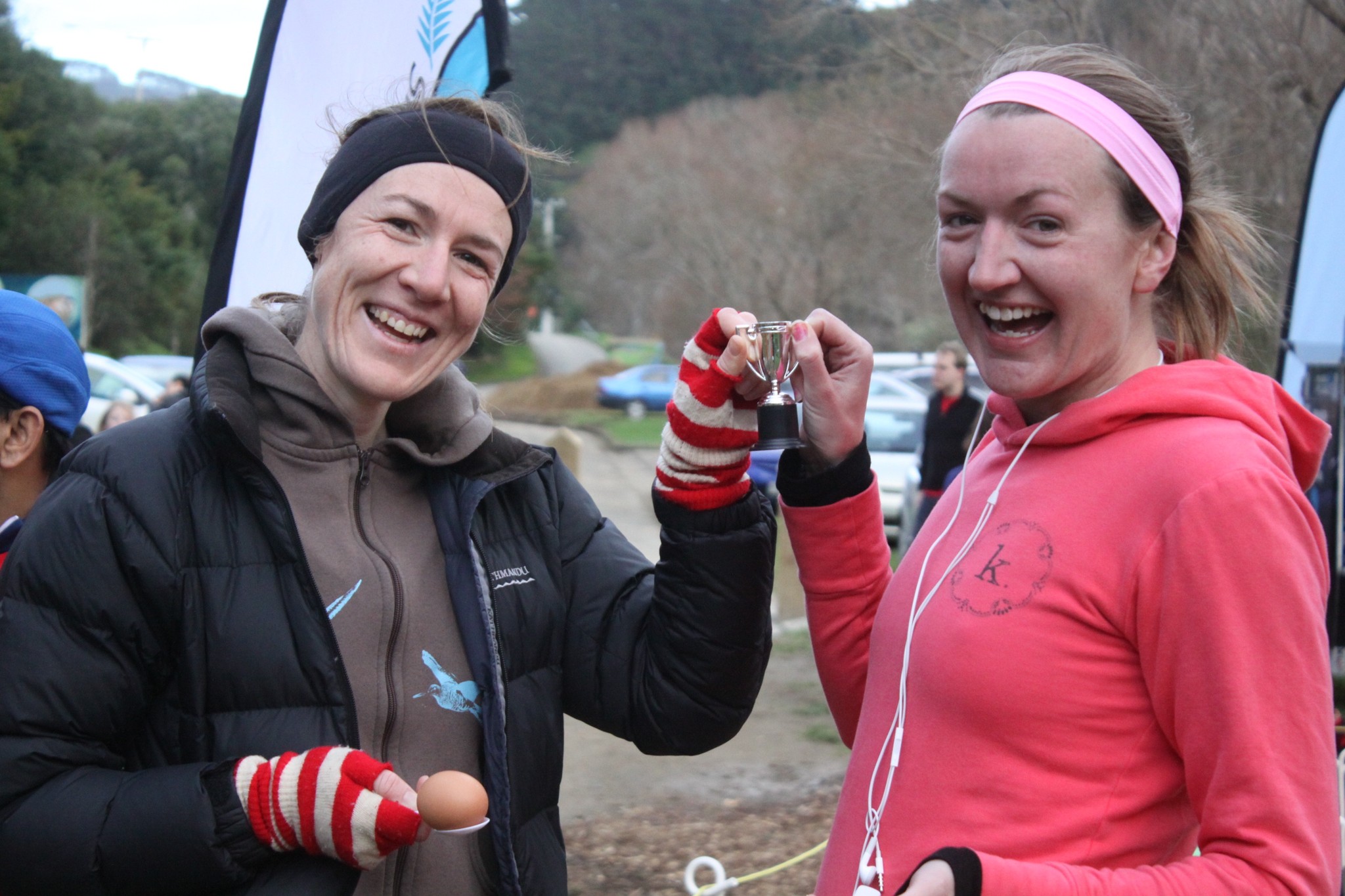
415;810;490;835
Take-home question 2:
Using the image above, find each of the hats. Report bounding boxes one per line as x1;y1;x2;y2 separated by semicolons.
0;290;91;435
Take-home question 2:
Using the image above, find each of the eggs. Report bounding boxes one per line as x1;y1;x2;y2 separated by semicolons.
417;770;488;831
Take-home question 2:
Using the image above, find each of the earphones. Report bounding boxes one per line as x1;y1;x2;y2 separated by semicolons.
858;837;878;887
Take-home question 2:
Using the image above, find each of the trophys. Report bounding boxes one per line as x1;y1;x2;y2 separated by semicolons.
736;319;808;452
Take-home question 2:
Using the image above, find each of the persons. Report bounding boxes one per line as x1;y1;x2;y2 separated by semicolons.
775;41;1342;896
907;339;986;544
0;89;777;896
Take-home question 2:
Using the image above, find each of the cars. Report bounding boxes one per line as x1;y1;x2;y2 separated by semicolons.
596;363;682;422
745;351;992;543
73;350;193;441
